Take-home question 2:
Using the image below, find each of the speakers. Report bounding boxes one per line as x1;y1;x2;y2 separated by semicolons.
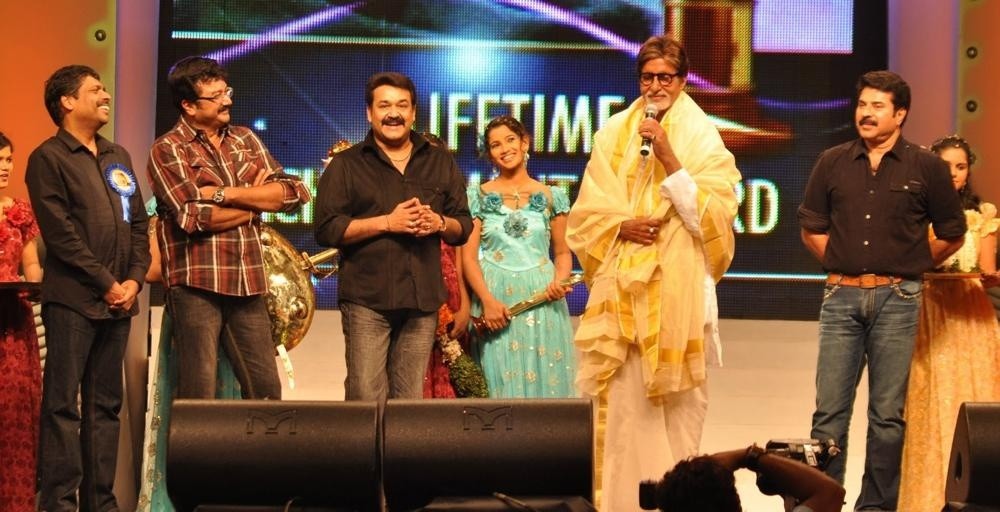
165;399;382;512
381;398;593;512
944;402;1000;512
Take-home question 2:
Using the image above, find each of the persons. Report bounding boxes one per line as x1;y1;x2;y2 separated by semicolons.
798;69;968;512
565;33;742;512
423;238;472;399
313;73;474;418
462;116;579;399
897;136;1000;512
145;55;312;400
135;193;244;512
655;445;846;512
0;131;45;512
24;65;153;512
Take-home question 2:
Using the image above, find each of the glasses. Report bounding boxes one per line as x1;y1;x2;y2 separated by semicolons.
638;69;684;87
194;86;234;102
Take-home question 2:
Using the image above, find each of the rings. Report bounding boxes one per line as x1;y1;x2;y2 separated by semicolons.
649;227;655;234
410;221;416;227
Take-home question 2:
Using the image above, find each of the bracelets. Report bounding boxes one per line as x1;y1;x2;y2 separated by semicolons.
386;215;390;232
437;215;446;233
745;442;768;468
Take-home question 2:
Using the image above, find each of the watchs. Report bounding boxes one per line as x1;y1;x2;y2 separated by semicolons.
212;185;228;207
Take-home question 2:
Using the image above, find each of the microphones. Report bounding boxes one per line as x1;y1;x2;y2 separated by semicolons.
639;104;659;156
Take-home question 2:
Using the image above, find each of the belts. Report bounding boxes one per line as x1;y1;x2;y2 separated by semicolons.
824;270;907;290
